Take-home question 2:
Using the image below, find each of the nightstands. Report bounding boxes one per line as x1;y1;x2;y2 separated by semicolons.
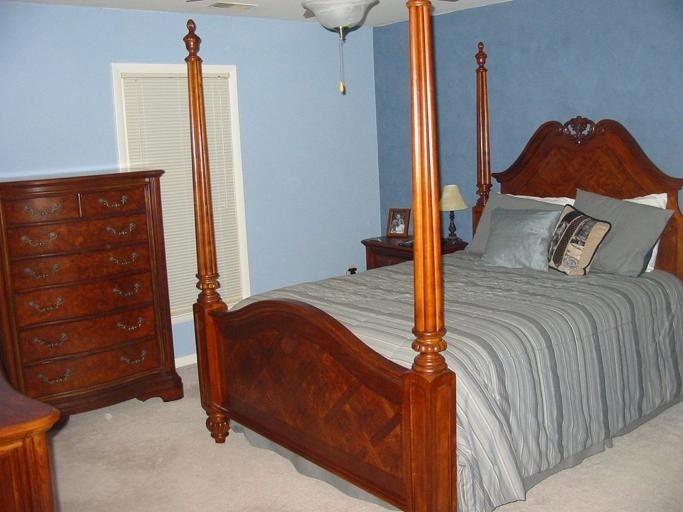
361;236;468;270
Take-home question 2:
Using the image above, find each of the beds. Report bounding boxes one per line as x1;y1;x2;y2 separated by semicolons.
183;0;683;512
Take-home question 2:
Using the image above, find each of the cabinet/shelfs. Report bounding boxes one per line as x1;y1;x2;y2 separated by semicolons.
0;169;183;415
0;366;60;512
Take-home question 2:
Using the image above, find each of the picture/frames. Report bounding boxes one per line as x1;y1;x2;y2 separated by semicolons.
386;208;411;238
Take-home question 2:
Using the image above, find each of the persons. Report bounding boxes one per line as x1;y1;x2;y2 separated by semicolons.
391;213;405;232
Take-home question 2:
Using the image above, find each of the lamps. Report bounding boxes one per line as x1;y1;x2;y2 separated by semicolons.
439;185;468;246
301;0;377;94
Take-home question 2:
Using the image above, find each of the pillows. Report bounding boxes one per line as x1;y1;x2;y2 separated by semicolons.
465;189;674;277
